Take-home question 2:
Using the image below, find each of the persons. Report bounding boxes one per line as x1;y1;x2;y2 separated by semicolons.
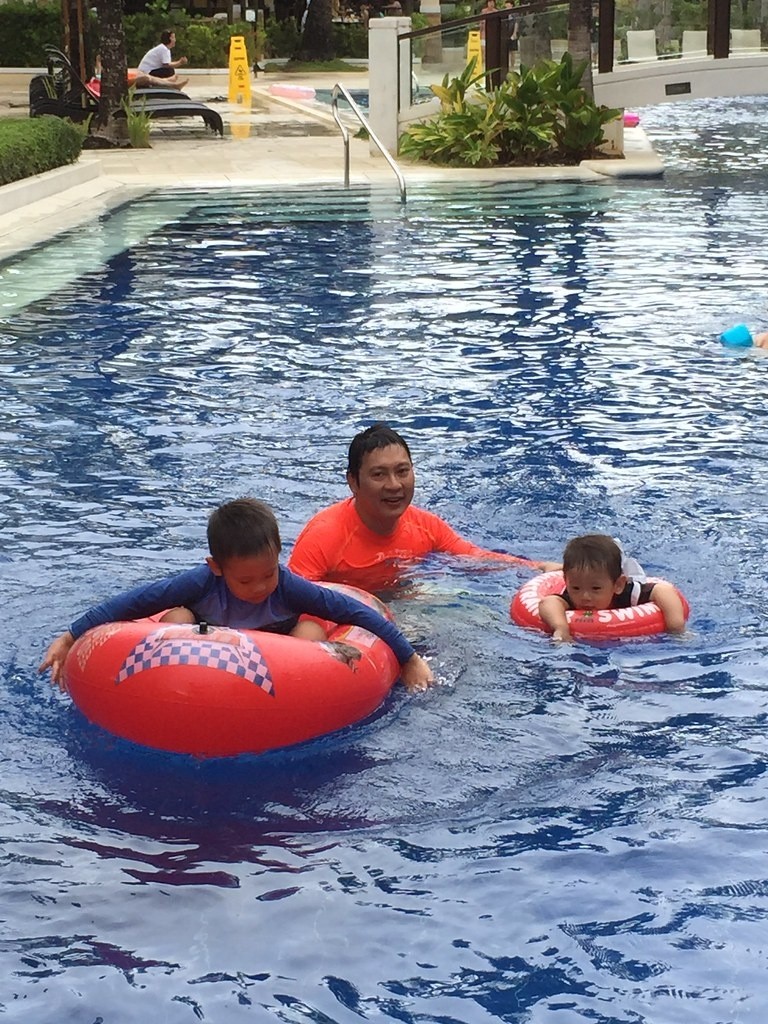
138;30;188;78
287;426;480;588
480;0;519;71
539;534;685;638
84;71;189;94
37;500;432;693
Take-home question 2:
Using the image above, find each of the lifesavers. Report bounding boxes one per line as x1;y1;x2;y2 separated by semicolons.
514;568;692;642
60;582;397;758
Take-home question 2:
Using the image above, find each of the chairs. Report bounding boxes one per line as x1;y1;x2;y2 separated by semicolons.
29;42;224;139
